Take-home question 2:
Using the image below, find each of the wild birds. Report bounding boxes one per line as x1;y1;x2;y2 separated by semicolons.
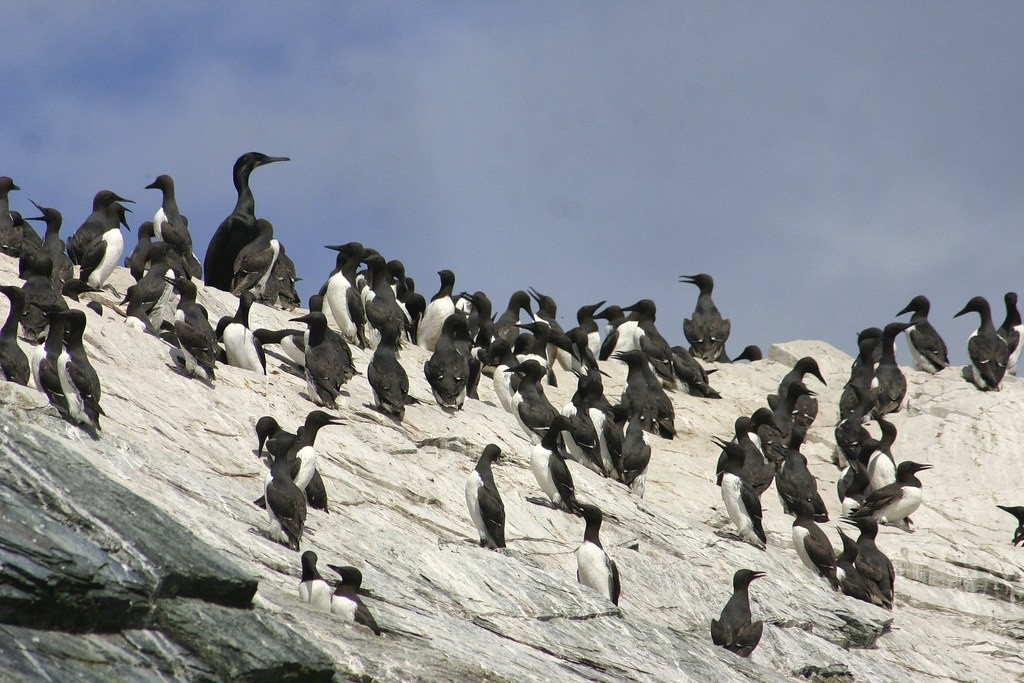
1;151;1024;657
